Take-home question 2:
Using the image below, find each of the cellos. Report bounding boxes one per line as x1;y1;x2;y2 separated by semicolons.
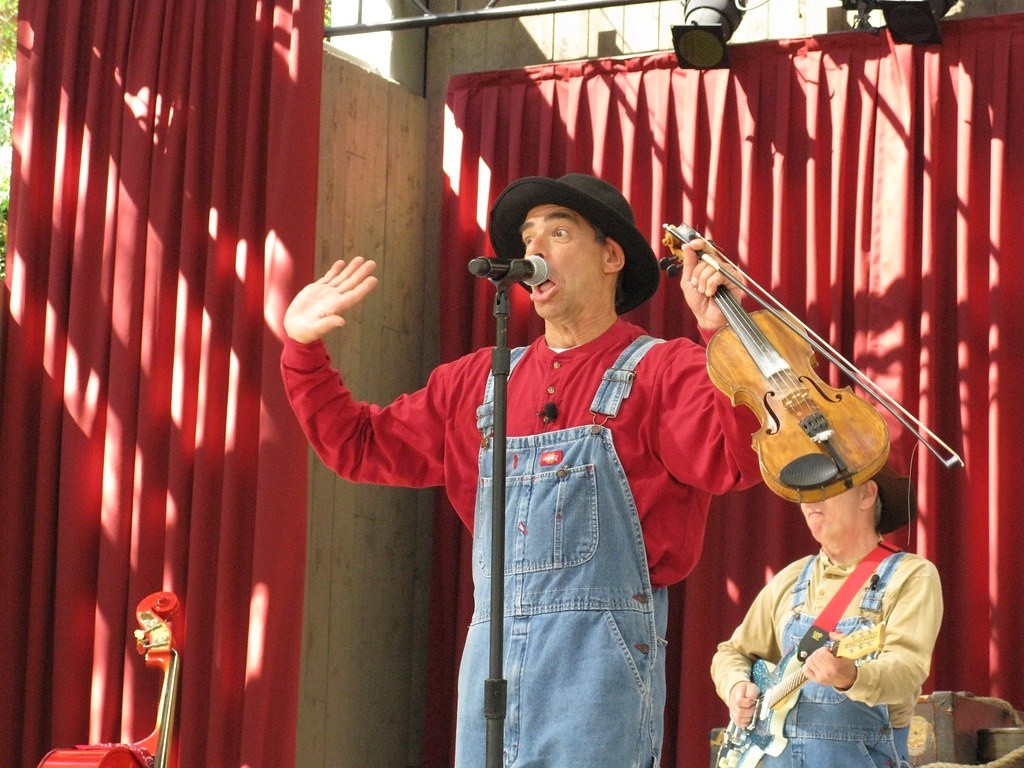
35;591;188;768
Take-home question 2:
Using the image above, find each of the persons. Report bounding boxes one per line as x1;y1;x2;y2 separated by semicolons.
710;463;944;768
283;173;764;768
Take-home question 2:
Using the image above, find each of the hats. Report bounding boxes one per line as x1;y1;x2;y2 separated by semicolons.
487;172;659;315
872;463;916;534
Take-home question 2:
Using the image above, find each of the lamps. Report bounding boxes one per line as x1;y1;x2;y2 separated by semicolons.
669;0;749;71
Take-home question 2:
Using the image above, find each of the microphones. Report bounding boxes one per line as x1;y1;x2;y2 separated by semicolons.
465;255;550;286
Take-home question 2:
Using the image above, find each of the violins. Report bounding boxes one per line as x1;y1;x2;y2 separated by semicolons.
658;220;892;504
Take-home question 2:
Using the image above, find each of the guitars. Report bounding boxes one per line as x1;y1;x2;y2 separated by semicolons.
715;622;887;768
